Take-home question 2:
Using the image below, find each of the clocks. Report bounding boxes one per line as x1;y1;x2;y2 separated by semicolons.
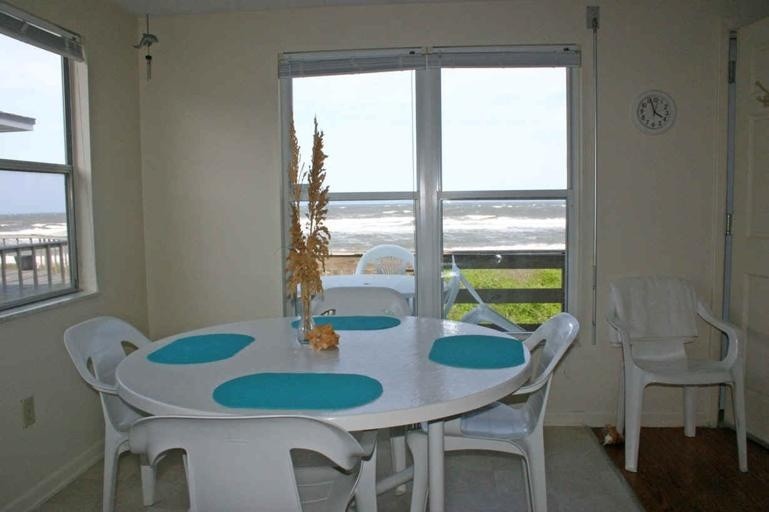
632;90;676;134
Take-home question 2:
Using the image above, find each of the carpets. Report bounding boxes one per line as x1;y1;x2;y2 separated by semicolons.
32;426;644;512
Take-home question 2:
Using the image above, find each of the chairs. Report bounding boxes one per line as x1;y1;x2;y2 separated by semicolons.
607;278;750;473
407;312;580;510
62;316;181;511
308;286;430;509
356;244;416;314
442;252;492;318
130;415;379;510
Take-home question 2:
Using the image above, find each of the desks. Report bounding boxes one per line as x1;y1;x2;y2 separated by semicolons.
110;313;531;511
295;273;449;297
